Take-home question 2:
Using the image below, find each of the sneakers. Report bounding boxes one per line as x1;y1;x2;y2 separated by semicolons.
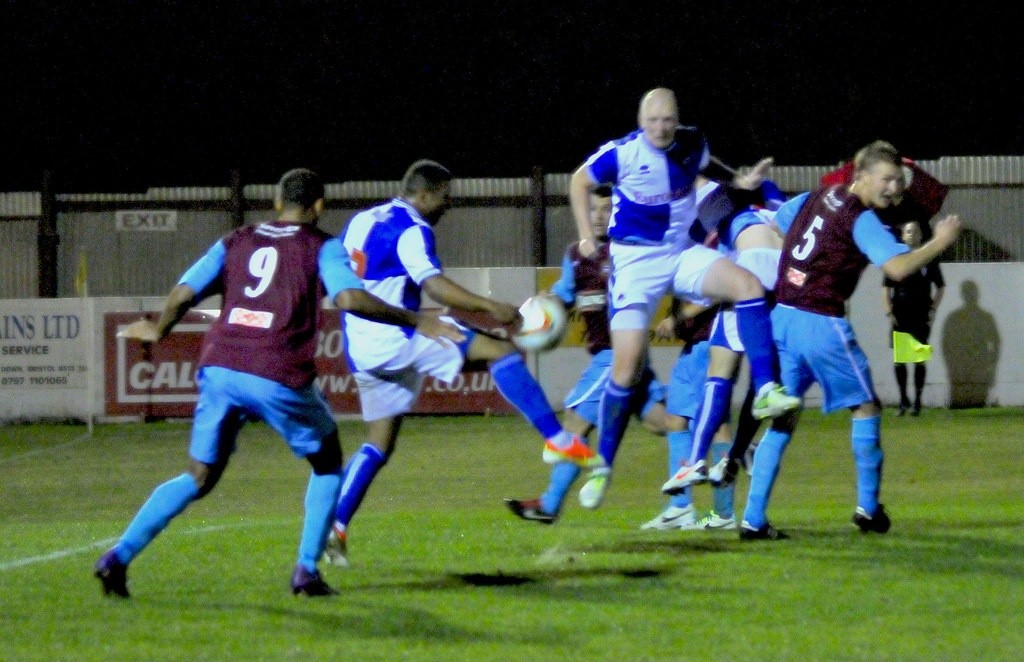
740;520;789;539
853;504;890;533
660;460;709;493
543;437;605;467
95;550;128;597
708;459;738;488
505;498;558;526
752;381;802;420
326;527;349;569
742;443;757;476
680;511;737;530
641;504;696;530
291;566;336;597
579;476;608;508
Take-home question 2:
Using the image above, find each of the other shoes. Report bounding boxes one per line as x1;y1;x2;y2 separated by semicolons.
896;397;922;417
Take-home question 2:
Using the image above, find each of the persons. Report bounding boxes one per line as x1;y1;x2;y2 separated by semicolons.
93;167;466;600
503;87;962;541
739;140;962;542
323;158;611;566
569;87;803;510
879;221;948;419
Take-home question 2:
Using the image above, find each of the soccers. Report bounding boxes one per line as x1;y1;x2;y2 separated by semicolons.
512;294;569;352
